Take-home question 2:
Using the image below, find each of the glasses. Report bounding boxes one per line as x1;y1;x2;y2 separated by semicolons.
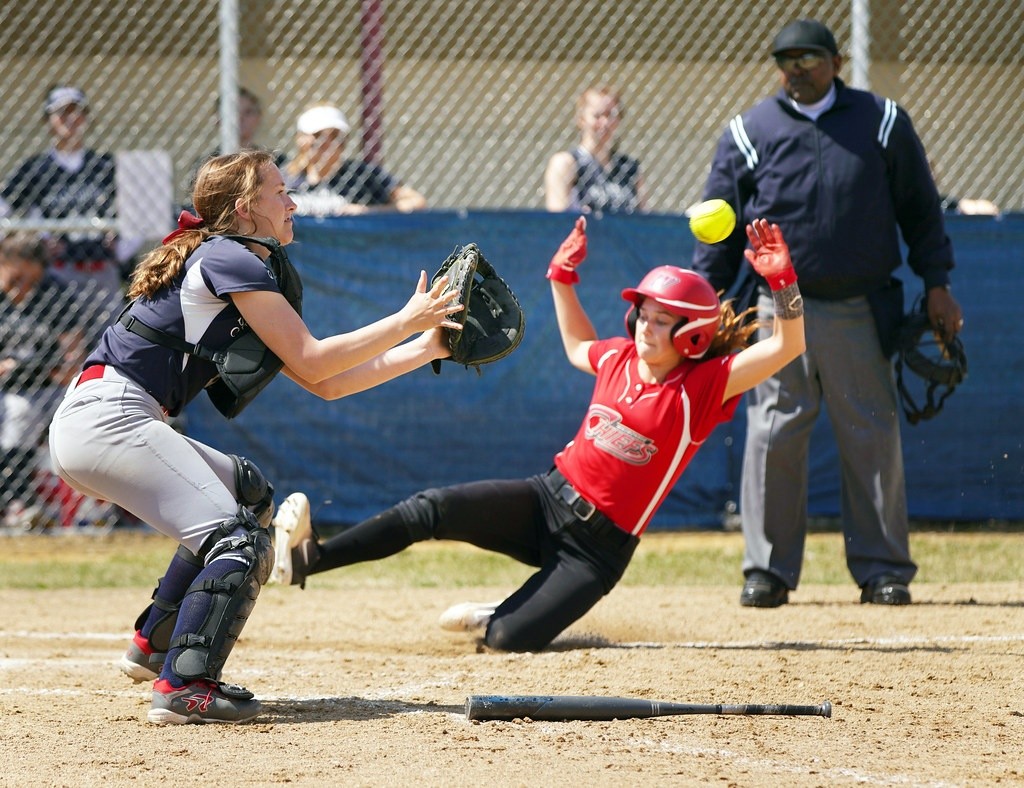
777;52;835;70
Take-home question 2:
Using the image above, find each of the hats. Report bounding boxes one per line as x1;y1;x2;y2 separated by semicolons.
297;105;349;135
44;83;89;116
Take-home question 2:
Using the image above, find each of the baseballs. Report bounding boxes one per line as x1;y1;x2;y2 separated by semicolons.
689;198;738;245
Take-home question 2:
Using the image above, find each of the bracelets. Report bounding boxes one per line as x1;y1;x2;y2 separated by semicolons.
772;283;804;319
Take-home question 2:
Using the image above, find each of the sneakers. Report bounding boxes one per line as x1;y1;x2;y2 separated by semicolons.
121;629;168;681
147;684;261;725
273;492;320;591
440;601;501;631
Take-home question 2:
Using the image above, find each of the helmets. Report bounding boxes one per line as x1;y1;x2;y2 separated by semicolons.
621;266;722;359
770;17;838;57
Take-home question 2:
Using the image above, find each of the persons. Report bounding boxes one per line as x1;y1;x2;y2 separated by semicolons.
213;87;288;165
693;22;963;607
940;194;996;215
48;152;524;722
0;85;117;529
546;85;645;212
273;214;806;654
284;102;426;209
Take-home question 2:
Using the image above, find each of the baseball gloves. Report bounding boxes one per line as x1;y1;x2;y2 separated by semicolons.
426;241;528;379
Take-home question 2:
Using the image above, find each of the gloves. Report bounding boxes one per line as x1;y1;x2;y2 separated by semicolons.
544;214;588;285
743;218;798;293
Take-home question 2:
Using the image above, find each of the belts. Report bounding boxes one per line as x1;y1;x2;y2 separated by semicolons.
550;468;628;547
75;365;103;384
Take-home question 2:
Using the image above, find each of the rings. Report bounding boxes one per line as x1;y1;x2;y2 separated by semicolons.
959;320;962;325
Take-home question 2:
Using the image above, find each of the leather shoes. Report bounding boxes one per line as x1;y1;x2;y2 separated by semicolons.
740;573;788;606
861;580;910;605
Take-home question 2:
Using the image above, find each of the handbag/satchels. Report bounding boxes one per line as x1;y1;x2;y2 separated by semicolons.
868;277;905;360
